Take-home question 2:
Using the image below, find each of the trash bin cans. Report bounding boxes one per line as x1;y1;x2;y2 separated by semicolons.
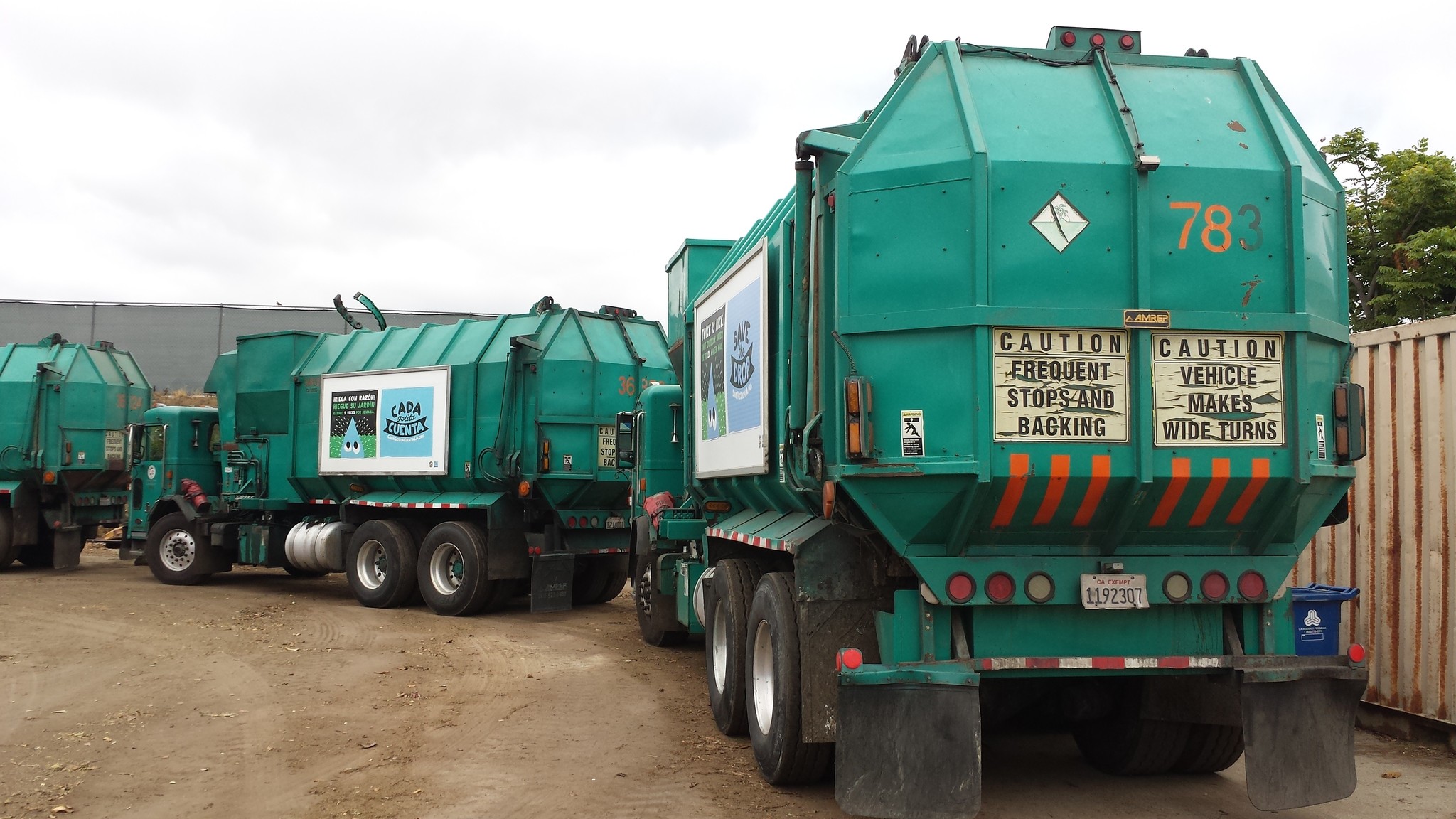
1286;581;1359;656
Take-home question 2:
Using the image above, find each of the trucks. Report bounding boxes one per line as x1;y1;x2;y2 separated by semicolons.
614;24;1372;819
122;289;676;619
1;333;156;574
